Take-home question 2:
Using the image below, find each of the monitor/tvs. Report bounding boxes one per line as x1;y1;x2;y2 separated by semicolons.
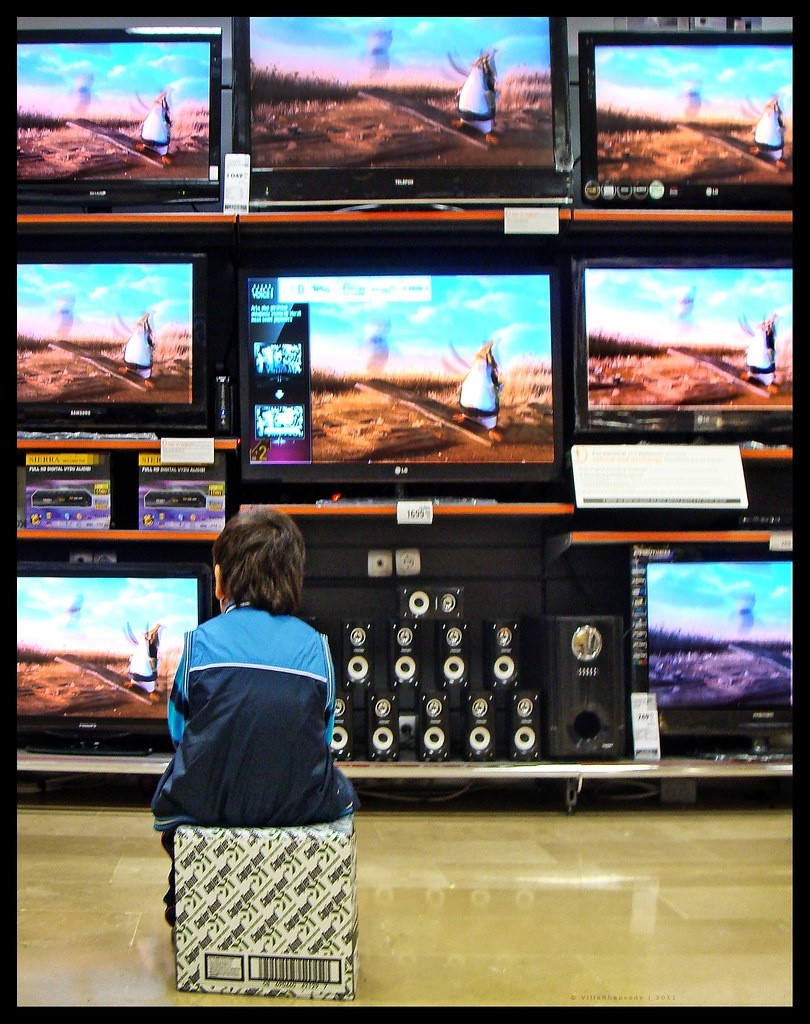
631;546;793;762
17;254;795;506
15;16;793;215
15;560;214;755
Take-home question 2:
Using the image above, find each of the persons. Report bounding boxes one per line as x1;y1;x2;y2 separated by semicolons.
149;507;353;948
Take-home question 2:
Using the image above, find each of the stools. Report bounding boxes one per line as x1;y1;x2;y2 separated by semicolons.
175;812;357;1000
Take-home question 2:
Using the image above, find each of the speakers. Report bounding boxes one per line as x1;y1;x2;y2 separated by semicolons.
330;585;628;766
